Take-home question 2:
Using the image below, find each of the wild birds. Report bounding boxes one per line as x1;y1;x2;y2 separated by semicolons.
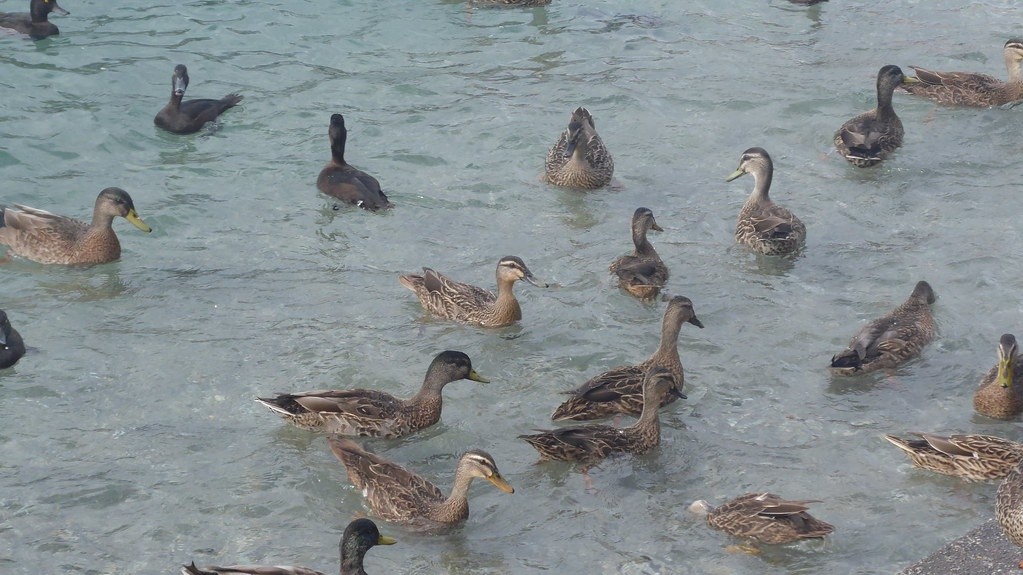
609;207;674;306
179;518;397;575
514;294;704;463
0;186;153;266
833;65;919;169
689;492;837;554
154;64;244;144
399;255;548;328
830;281;1023;568
727;147;807;257
0;0;71;41
256;351;490;439
891;39;1023;117
541;105;623;194
318;113;396;213
0;309;26;370
324;437;515;536
464;0;553;23
